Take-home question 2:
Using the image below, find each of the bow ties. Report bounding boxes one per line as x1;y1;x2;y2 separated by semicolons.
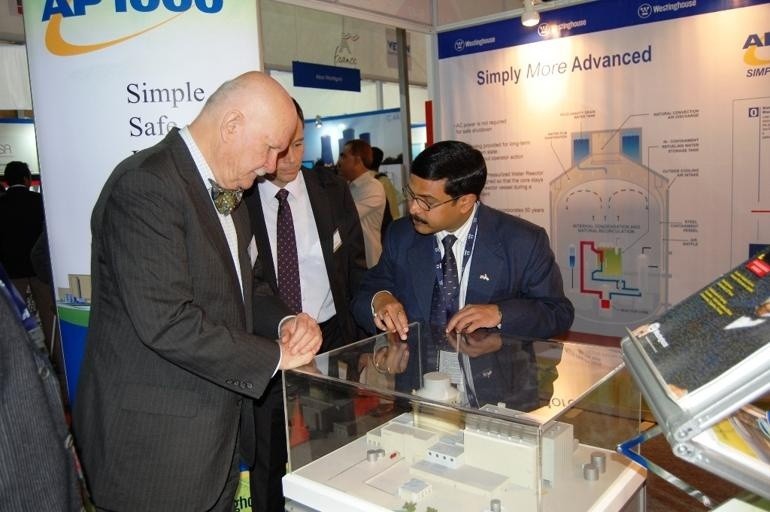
207;178;243;217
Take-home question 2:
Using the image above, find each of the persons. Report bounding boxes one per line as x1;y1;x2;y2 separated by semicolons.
350;138;576;340
68;69;326;512
336;139;398;419
0;160;56;354
28;225;55;317
627;295;769;400
241;95;369;512
371;320;566;419
368;146;401;222
0;282;88;512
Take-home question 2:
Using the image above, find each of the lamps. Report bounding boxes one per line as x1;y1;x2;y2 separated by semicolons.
314;115;322;128
519;0;540;26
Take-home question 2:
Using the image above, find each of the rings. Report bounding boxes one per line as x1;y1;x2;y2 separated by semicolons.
382;310;389;318
398;310;402;315
399;368;405;373
386;367;391;372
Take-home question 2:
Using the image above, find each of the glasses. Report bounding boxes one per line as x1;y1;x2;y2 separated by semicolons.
401;183;461;212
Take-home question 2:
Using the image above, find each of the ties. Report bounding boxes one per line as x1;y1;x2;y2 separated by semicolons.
274;189;304;313
429;234;463;350
